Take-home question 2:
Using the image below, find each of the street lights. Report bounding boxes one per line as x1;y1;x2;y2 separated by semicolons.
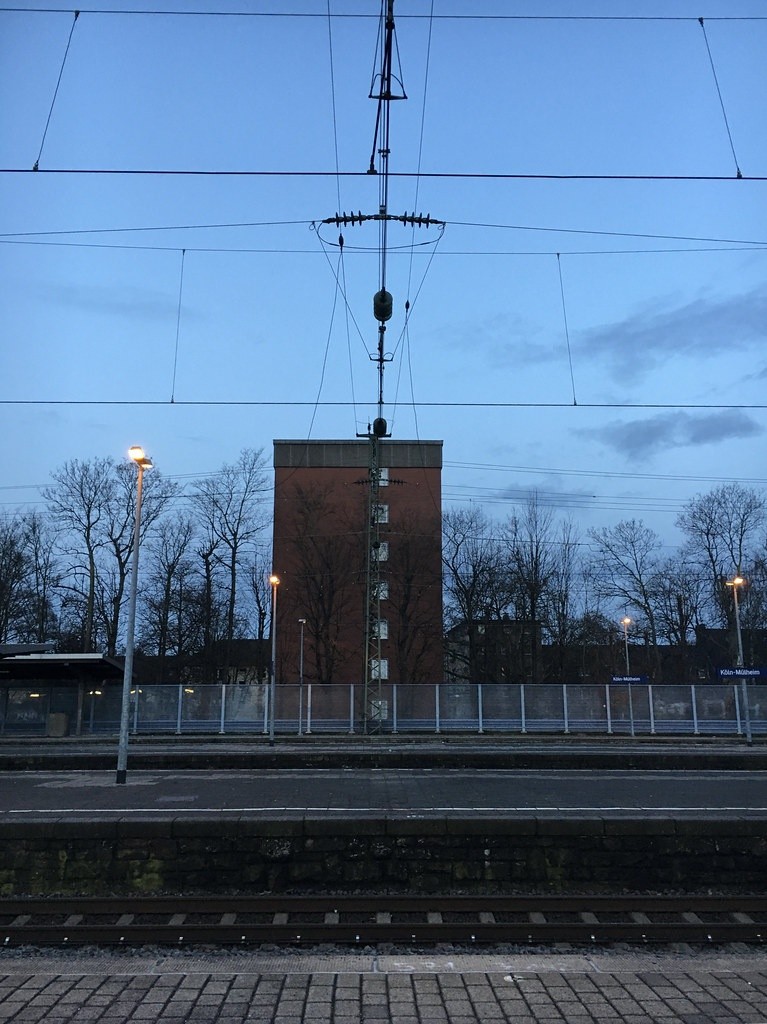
297;617;306;736
622;618;636;738
111;445;156;785
268;575;280;748
727;577;756;745
